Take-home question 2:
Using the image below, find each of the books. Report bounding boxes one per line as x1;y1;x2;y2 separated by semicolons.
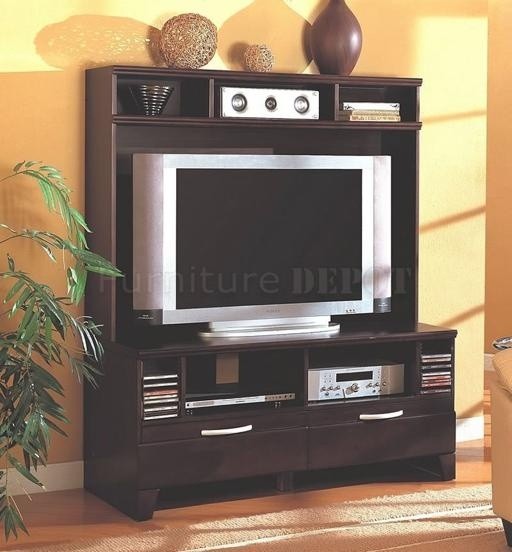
338;101;402;122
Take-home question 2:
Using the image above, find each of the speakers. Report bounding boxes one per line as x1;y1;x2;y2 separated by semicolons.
216;86;320;120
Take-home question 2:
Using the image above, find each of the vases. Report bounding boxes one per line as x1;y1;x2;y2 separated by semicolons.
307;0;362;76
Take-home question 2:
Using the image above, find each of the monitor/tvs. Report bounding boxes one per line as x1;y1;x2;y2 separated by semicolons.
132;153;392;337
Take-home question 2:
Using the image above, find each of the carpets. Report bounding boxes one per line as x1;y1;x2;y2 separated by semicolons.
1;480;508;552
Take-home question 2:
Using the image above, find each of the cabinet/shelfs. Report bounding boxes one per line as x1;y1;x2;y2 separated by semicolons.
82;65;458;523
